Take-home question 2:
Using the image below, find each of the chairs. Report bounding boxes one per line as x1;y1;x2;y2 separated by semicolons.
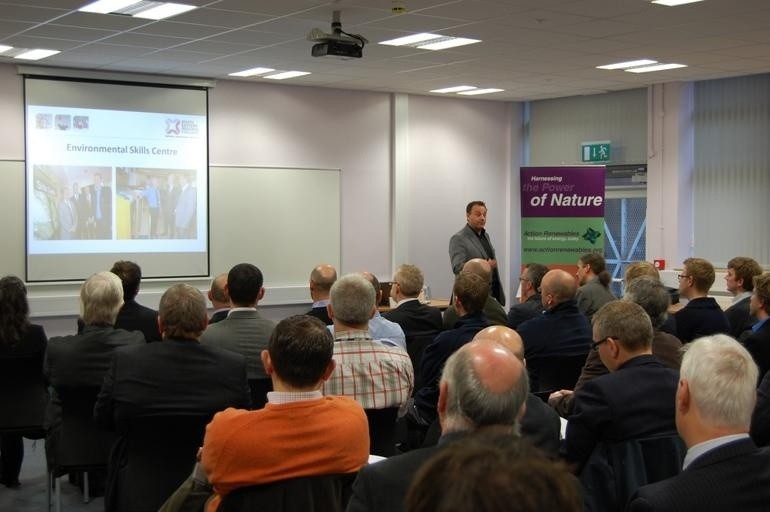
2;311;769;512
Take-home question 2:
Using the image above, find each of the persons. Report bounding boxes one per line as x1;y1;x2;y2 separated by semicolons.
449;200;508;304
33;167;197;239
0;253;770;512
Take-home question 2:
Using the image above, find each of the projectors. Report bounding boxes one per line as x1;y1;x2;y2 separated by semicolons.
312;40;363;58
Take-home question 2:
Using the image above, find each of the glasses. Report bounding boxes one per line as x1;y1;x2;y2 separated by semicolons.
591;337;620;353
678;275;690;279
